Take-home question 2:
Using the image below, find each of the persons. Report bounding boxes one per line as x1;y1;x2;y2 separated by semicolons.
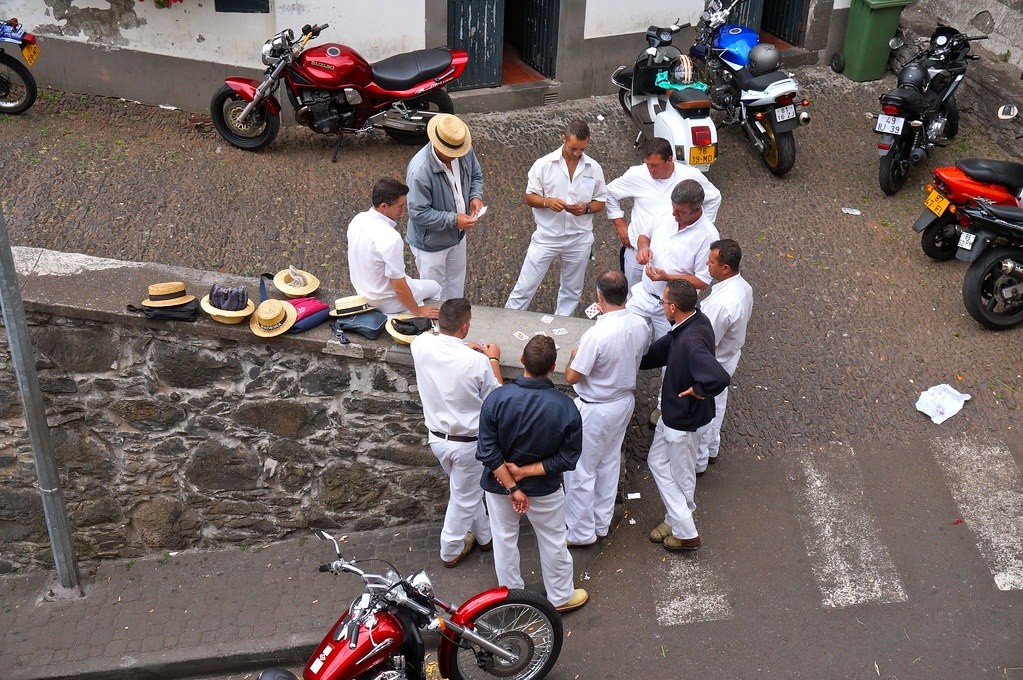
475;335;590;613
348;179;442;319
605;138;722;295
505;118;607;316
626;179;720;429
404;113;485;301
639;279;730;551
696;239;754;476
411;298;503;567
564;269;652;546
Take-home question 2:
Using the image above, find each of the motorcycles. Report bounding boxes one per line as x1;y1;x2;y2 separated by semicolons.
610;19;719;173
687;0;811;178
209;23;470;164
259;529;563;680
862;26;988;196
911;157;1023;262
0;18;43;115
954;197;1023;330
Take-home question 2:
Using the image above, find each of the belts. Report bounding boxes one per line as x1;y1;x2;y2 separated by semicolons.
430;429;478;442
579;397;597;404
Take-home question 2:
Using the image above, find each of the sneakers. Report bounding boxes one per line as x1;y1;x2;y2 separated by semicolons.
651;294;661;300
663;534;703;550
555;588;588;612
444;529;474;567
648;520;673;543
481;540;493;552
565;529;597;545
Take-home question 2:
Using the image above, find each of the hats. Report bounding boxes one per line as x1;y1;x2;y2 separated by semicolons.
273;269;320;299
329;296;374;316
385;313;431;345
141;282;195;307
250;299;297;338
427;113;472;157
200;295;255;325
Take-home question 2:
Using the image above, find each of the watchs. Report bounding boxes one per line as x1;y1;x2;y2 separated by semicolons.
584;204;591;214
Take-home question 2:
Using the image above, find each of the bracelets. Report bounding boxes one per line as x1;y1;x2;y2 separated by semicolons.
505;485;520;494
489;357;500;362
544;197;551;208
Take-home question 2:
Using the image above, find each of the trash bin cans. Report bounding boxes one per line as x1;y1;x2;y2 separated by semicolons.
833;1;917;83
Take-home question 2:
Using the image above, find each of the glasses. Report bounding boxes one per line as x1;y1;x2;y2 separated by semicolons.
659;300;679;309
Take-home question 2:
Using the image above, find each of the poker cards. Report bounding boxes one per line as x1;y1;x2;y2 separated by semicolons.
648;260;657;275
555;342;561;351
552;328;569;336
512;331;530;341
584;301;601;319
476;336;487;353
472;205;487;219
534;331;548;337
540;314;555;325
566;196;579;206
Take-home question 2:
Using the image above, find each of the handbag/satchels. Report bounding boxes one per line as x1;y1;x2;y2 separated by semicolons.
332;309;387;342
260;273;331;334
209;283;248;311
126;299;200;322
391;317;432;335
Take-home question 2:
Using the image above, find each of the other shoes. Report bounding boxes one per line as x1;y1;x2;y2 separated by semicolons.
696;472;706;477
710;456;719;464
649;408;662;424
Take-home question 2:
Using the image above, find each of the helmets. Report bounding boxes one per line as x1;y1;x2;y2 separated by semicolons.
668;55;694;85
748;43;781;75
898;63;924;93
